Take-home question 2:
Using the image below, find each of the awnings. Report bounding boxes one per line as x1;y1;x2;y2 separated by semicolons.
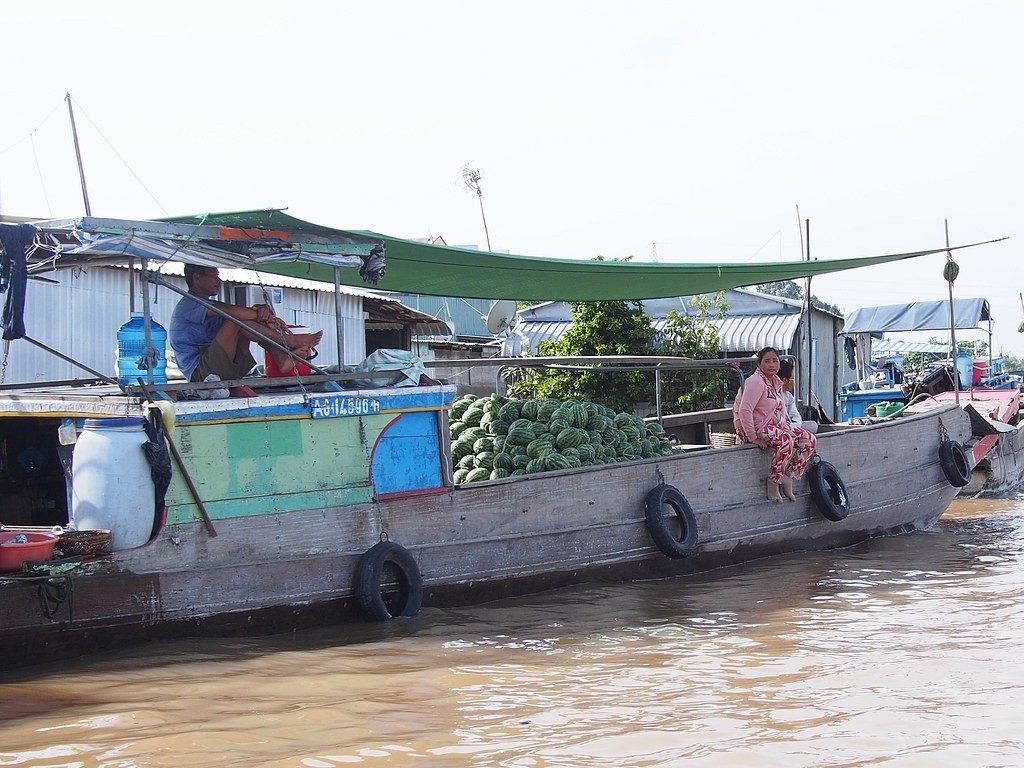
501;313;800;355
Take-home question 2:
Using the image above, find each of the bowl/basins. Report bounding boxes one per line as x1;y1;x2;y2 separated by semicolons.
858;380;872;390
0;532;59;572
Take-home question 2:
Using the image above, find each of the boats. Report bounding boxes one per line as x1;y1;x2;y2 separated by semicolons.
0;206;1019;672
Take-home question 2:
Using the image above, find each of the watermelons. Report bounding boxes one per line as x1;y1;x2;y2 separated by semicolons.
446;394;685;486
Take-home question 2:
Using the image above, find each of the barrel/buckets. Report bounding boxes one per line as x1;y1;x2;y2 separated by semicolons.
973;359;992;386
71;419;157;552
874;402;904;417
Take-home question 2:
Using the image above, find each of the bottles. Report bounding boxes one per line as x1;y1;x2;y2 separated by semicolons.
116;312;167;393
956;353;973;390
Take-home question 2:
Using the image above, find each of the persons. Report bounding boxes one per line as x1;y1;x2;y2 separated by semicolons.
169;264;323;382
775;360;818;434
732;347;816;502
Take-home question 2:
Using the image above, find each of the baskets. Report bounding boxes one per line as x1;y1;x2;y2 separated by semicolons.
707;422;737;450
56;529;112;556
